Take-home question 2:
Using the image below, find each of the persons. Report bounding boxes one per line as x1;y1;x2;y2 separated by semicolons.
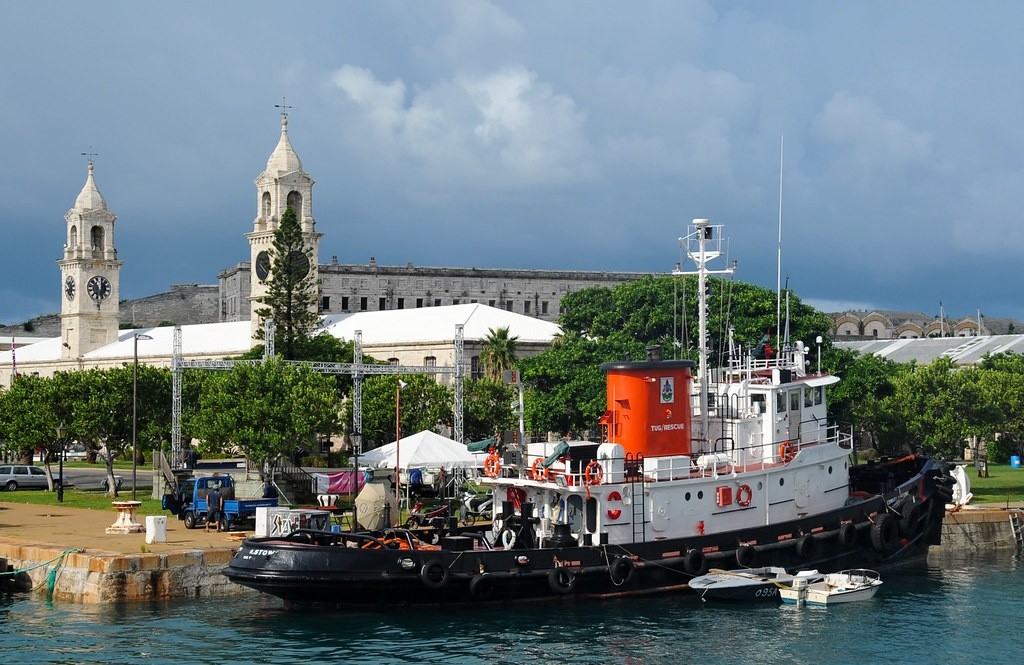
435;467;448;486
409;470;424;486
205;484;223;533
387;467;400;489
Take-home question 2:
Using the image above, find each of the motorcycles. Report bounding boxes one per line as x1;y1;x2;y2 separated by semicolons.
406;500;450;530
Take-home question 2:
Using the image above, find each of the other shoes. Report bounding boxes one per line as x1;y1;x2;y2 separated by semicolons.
203;529;209;533
216;530;220;533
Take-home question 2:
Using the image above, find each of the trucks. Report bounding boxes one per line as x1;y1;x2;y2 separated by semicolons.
161;475;278;532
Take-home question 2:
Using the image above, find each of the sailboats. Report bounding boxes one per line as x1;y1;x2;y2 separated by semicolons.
220;132;959;612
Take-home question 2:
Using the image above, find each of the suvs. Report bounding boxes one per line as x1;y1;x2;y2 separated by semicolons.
0;464;68;491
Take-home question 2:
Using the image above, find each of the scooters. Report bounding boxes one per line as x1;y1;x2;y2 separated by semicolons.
460;489;496;521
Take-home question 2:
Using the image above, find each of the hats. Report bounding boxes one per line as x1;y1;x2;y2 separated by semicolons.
213;484;220;489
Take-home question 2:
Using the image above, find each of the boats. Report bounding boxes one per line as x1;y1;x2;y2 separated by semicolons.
688;566;825;603
778;569;883;606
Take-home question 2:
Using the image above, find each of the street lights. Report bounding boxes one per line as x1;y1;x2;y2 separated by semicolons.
132;333;154;500
55;422;68;503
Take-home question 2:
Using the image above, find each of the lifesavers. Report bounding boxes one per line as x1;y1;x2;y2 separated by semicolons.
683;548;707;576
609;556;636;588
796;534;817;561
548;565;577;596
869;512;903;555
837;522;856;549
778;440;794;462
483;454;501;479
530;458;550;482
420;558;451;589
736;484;752;507
901;501;929;540
468;572;496;602
585;461;604;485
607;490;623;520
735;543;759;569
